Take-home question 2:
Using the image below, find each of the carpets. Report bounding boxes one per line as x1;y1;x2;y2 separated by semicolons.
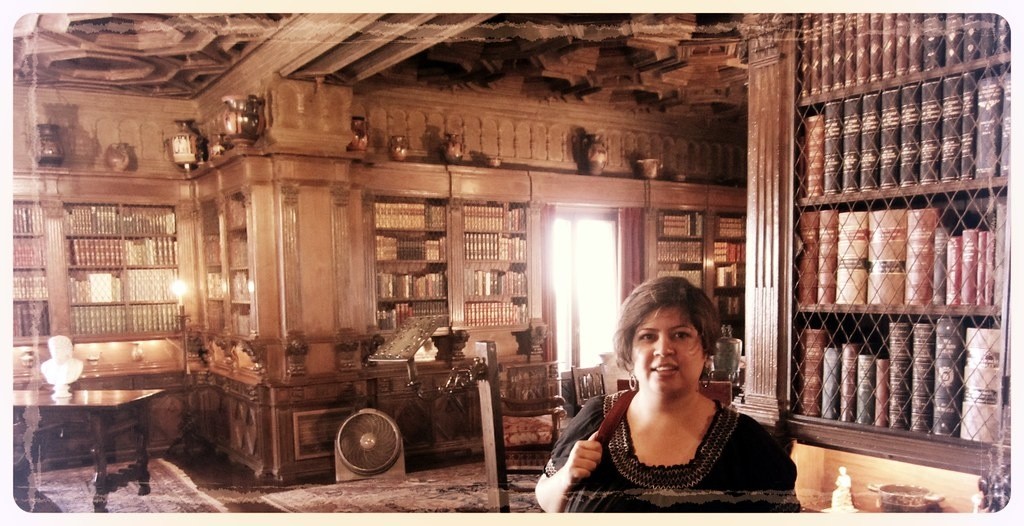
260;461;547;513
12;459;229;514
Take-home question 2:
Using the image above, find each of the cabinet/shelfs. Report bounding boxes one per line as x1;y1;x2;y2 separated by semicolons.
62;194;181;344
15;194;50;347
793;13;1012;479
499;361;559;401
462;196;531;333
199;187;251;342
654;207;708;294
14;362;207;467
366;188;454;337
710;205;747;322
208;365;270;483
269;368;370;486
371;357;484;467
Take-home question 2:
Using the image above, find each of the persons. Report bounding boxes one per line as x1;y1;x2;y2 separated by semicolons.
534;276;802;513
40;335;84;386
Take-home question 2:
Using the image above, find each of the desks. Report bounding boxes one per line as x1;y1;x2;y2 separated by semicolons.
13;389;165;514
791;444;986;513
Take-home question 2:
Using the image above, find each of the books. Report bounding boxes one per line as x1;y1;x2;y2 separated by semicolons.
370;195;531;329
788;14;1011;448
654;210;746;325
10;196;251;344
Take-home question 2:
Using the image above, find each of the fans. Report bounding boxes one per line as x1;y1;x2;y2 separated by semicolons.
332;408;407;485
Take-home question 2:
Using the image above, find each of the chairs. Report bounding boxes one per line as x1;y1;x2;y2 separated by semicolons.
617;380;731;408
475;340;568;513
571;364;610;418
